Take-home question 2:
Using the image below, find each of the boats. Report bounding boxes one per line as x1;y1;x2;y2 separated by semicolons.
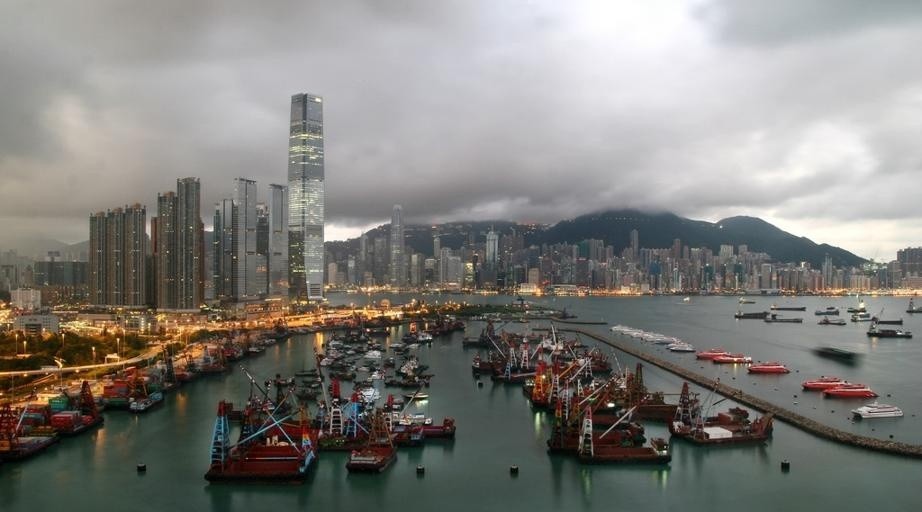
463;317;775;465
733;311;770;320
291;314;466;412
343;443;397;474
0;401;60;461
738;297;756;305
812;295;922;367
802;375;852;391
102;325;294;412
748;361;790;375
823;383;879;400
763;314;804;324
204;363;321;483
770;305;807;312
851;400;905;419
51;380;103;436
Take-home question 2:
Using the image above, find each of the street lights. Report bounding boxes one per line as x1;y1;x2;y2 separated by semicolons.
5;322;97;384
